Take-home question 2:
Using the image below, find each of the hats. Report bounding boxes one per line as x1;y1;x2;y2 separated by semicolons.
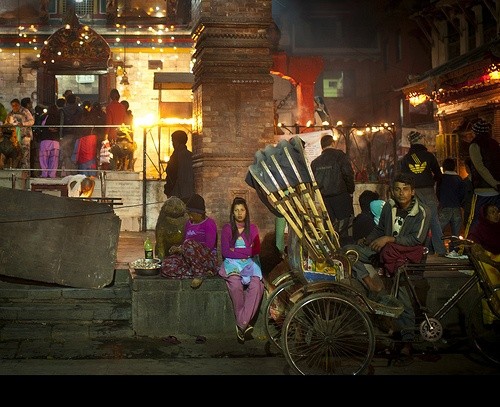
408;131;424;147
186;194;206;215
471;117;492;137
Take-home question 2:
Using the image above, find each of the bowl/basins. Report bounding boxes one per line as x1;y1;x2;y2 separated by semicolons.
136;258;162;265
134;263;161;276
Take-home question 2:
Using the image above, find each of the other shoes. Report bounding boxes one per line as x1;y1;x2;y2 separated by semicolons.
207;270;215;280
191;278;203;288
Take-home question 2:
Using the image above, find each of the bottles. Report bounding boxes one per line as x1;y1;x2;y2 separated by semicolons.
143;235;153;258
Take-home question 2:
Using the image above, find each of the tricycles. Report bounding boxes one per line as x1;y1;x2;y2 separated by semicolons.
245;135;500;374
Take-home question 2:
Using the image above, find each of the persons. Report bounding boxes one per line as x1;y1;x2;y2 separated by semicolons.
310;134;356;248
470;202;500;298
61;174;94;198
355;173;431;276
464;116;500;241
164;130;195;202
429;158;466;251
159;193;219;288
1;86;137;179
219;197;265;341
353;190;381;240
401;129;448;256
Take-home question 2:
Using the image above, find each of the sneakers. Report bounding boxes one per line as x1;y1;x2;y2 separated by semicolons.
235;325;253;342
